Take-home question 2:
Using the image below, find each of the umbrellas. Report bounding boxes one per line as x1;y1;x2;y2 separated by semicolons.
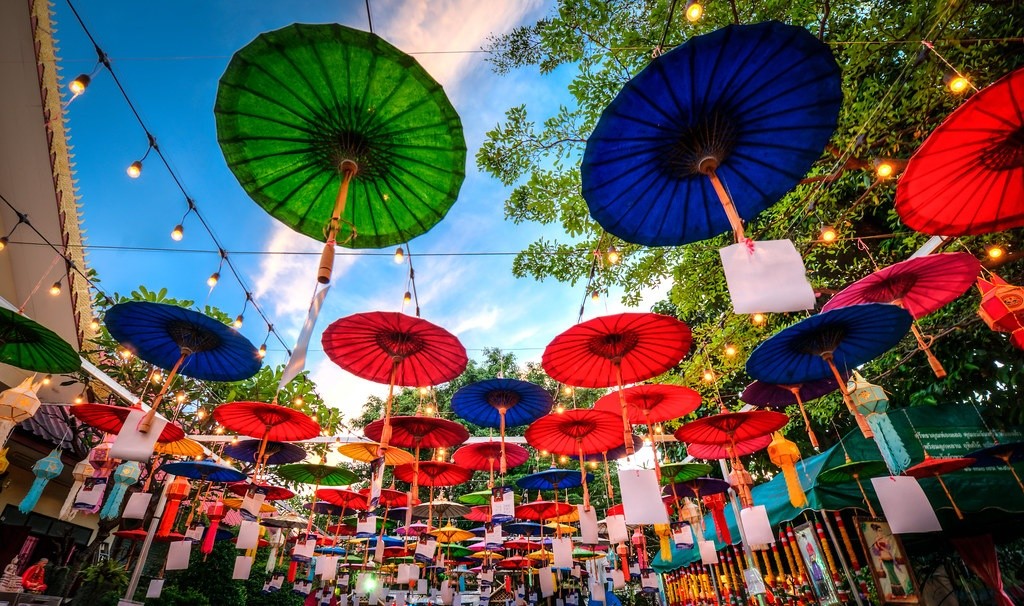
0;280;1024;578
888;68;1024;237
814;250;981;379
203;18;477;283
567;13;845;253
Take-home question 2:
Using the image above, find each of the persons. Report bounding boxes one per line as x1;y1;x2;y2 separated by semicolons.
21;558;48;592
1;556;19;593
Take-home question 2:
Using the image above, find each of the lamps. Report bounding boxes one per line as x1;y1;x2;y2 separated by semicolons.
127;145;152;178
928;56;966;91
815;211;834;241
607;235;618;262
68;59;101;94
865;142;892;176
395;245;404;261
207;257;222;286
92;301;108;327
685;0;703;20
171;208;192;241
259;329;271;352
0;219;22;251
235;298;249;328
294;383;333;435
50;266;71;296
403;274;412;303
